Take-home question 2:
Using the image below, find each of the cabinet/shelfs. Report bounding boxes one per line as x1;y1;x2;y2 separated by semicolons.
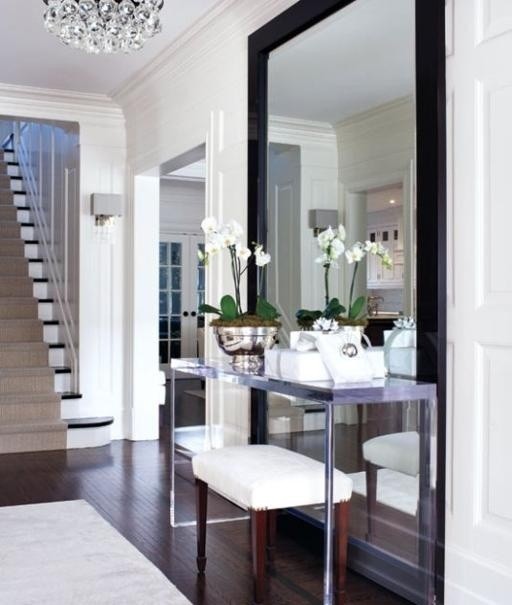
367;224;403;287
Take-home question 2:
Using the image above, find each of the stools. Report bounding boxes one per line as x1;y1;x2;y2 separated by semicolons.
189;444;354;605
361;431;420;543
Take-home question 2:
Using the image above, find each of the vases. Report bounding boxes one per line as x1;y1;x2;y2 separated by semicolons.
290;324;362;351
212;327;279;355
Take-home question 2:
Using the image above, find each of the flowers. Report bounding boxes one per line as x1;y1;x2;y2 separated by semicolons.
345;241;393;323
294;224;344;335
194;216;281;327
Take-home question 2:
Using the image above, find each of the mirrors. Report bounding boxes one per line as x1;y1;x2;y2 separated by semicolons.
247;0;444;604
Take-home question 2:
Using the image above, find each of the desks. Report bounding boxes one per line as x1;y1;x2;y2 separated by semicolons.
169;358;439;605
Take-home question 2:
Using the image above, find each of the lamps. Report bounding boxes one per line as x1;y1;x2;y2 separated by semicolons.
42;0;164;56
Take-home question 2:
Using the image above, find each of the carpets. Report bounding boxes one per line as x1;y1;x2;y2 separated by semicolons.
345;468;419;518
0;499;193;605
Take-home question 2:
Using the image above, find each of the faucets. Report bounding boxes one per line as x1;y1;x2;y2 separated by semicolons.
368;296;384;316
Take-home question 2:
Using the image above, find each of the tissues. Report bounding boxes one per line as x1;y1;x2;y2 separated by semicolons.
278;332;333;382
